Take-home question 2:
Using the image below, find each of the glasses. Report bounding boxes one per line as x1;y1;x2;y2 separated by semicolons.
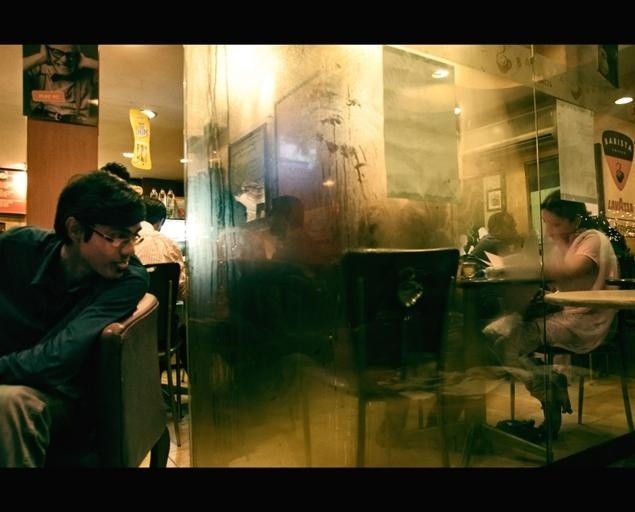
82;223;144;248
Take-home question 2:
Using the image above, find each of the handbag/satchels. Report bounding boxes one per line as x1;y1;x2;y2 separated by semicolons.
521;287;564;322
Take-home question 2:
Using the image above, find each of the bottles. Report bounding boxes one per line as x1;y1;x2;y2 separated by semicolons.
149;187;175;220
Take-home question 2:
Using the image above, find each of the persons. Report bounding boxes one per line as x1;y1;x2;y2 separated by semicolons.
101;162;130;185
0;167;149;472
197;177;625;464
129;192;193;373
21;44;99;126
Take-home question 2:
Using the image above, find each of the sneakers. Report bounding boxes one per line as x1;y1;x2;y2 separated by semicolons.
495;419;557;443
